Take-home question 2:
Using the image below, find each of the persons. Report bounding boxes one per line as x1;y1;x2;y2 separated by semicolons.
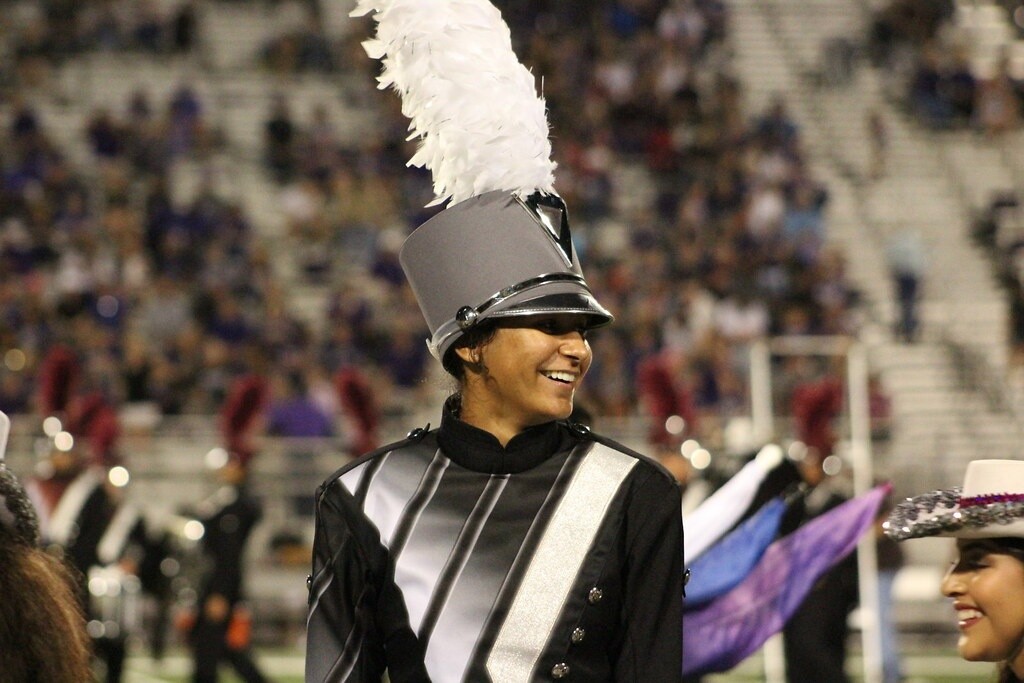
0;3;1024;682
883;460;1023;682
308;186;687;683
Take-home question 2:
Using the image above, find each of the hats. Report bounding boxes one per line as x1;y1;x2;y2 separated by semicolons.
882;460;1024;544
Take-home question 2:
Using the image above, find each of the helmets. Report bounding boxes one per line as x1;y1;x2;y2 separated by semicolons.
348;0;613;380
